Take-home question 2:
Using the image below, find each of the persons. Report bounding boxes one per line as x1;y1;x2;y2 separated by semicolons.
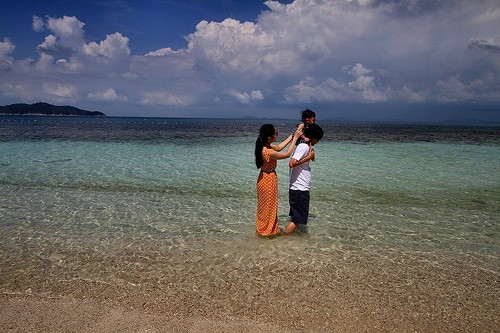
291;109;317;162
254;123;303;237
284;124;324;235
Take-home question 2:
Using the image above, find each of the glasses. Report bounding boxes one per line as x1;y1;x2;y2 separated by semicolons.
273;132;278;137
